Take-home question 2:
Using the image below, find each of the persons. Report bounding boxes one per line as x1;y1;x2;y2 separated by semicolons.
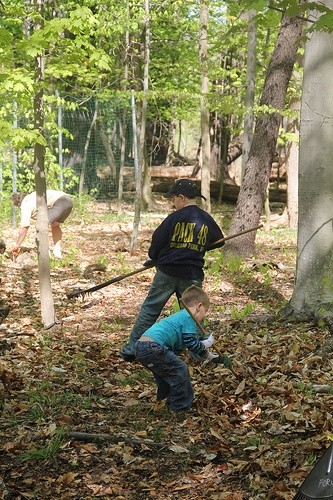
135;284;231;413
9;190;74;261
119;179;224;362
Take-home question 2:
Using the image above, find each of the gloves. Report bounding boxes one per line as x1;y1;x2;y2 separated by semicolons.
200;333;214;350
143;259;154;267
206;352;232;368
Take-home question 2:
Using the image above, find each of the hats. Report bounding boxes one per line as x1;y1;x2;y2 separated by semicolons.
163;179;206;201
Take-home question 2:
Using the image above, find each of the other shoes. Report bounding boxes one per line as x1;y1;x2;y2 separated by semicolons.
175;411;199;423
